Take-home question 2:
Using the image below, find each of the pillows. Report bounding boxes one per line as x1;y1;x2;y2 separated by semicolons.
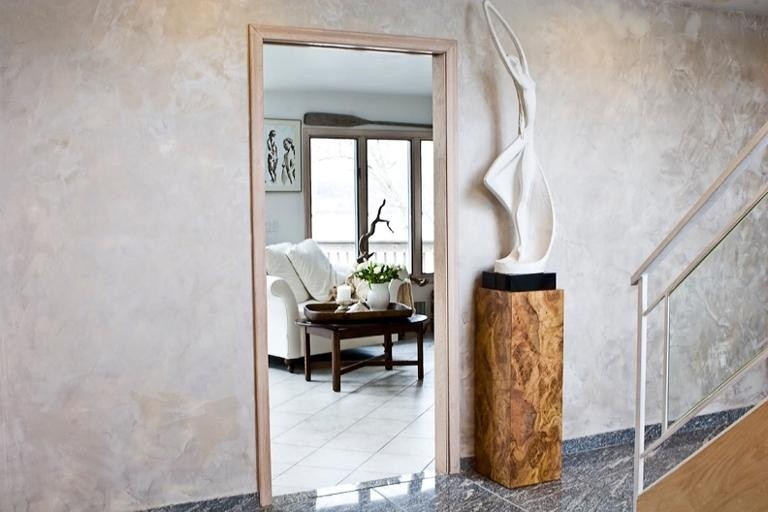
265;237;347;305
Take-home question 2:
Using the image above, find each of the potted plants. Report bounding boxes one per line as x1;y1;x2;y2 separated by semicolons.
349;260;405;310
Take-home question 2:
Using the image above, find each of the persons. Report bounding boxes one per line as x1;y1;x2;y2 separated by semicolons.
266;130;297;184
483;0;537;258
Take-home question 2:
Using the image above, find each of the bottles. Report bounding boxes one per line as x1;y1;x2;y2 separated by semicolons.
374;252;409;280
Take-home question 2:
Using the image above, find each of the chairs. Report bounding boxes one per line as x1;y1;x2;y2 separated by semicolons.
265;240;416;373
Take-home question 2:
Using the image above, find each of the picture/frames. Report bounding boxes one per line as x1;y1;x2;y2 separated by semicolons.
263;116;303;192
